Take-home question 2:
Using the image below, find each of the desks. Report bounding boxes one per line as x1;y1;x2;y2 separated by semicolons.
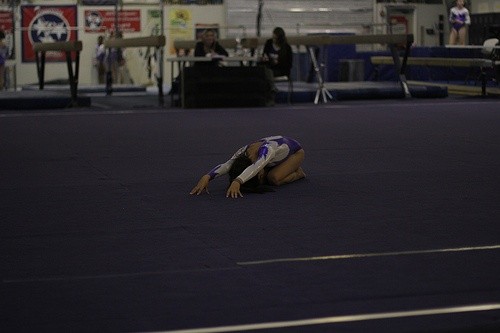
167;57;260;107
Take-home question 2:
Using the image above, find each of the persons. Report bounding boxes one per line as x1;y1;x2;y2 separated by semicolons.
193;29;230;68
0;30;10;92
93;35;107;84
256;27;295;108
189;135;305;199
448;0;471;47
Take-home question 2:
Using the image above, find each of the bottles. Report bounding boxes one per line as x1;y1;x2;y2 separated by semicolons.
236;41;242;56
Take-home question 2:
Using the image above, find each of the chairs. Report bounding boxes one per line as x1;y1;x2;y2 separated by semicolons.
275;71;294;104
